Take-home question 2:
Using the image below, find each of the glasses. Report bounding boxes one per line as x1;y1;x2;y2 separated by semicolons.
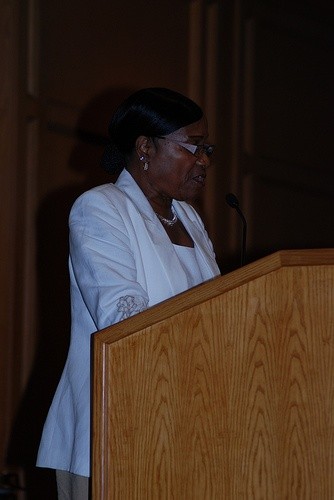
157;134;217;158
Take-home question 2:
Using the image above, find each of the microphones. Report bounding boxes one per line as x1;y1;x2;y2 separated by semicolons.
225;193;246;266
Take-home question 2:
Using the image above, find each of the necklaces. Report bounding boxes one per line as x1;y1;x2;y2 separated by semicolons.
150;207;178;226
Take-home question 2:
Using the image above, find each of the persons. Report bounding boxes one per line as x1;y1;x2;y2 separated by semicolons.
35;87;222;500
